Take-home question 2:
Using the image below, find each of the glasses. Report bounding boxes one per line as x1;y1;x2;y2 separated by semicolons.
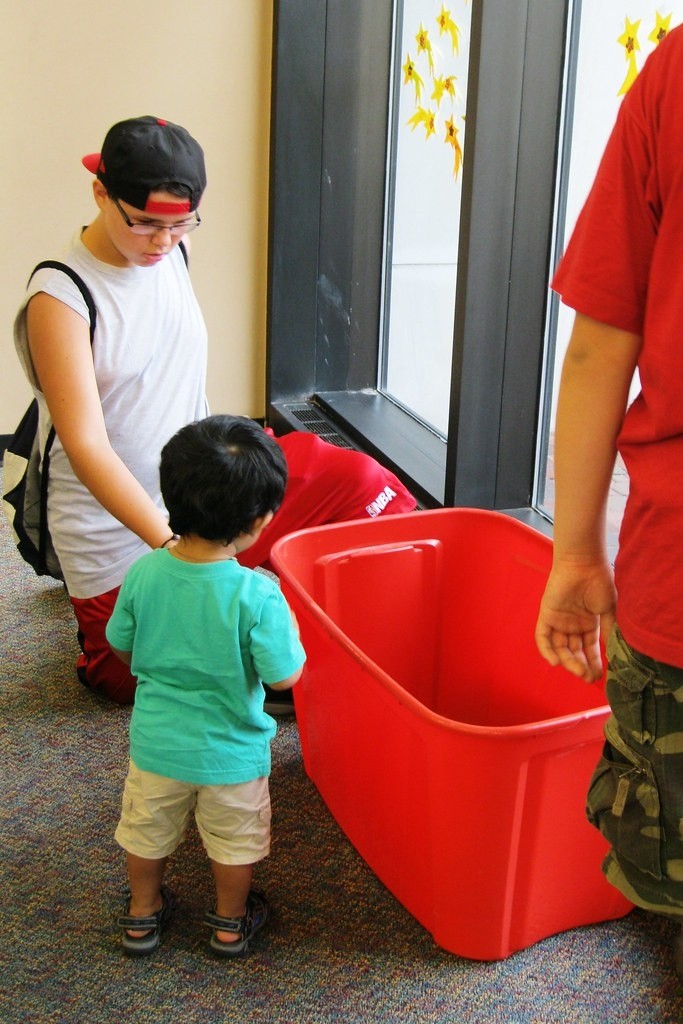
98;178;201;236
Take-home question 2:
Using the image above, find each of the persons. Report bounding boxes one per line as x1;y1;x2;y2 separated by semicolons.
104;415;307;958
535;32;683;916
13;115;207;698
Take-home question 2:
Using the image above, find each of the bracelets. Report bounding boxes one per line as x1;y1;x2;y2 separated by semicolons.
161;534;180;548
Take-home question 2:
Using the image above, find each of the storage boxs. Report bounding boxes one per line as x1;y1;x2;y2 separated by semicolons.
271;506;638;961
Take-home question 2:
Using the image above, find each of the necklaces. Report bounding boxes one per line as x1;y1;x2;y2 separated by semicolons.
170;545;235;561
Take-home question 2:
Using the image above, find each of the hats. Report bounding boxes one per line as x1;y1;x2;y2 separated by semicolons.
82;116;205;214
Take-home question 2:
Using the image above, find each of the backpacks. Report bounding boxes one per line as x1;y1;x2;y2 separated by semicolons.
3;241;189;581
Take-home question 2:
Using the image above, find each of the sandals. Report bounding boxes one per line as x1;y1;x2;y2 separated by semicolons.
203;890;267;956
118;885;169;954
